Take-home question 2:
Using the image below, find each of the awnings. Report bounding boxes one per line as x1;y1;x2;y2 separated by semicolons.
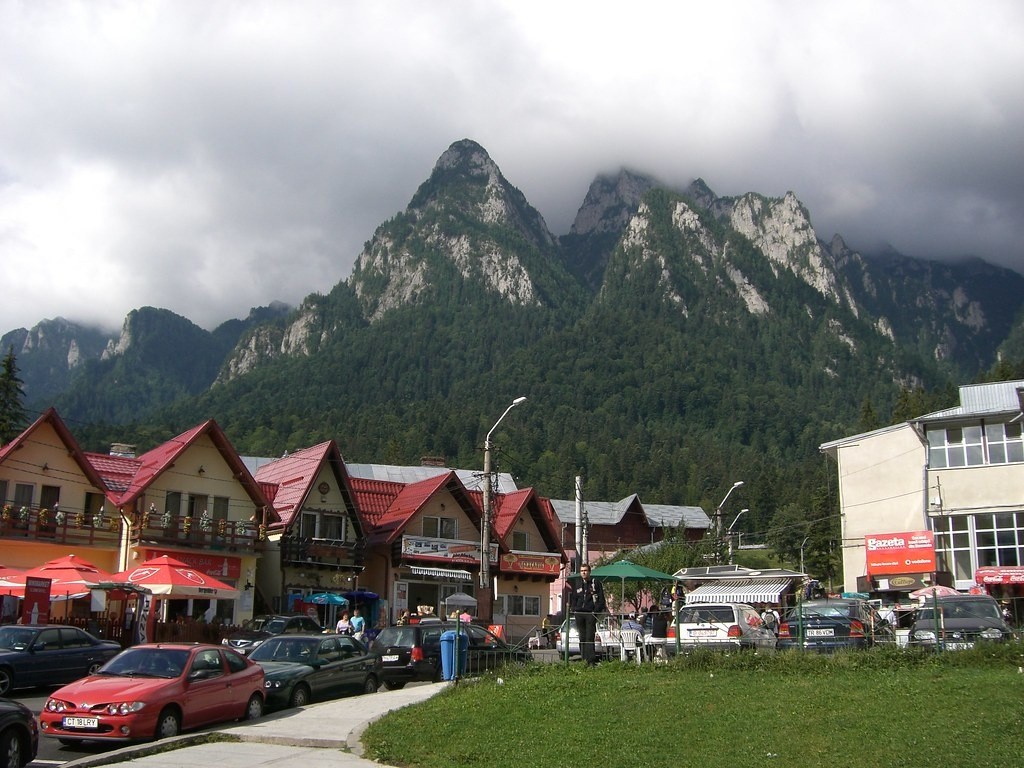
685;579;791;604
975;566;1024;584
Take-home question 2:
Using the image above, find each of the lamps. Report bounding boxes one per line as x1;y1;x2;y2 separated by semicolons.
43;462;48;474
245;579;255;592
198;465;205;477
520;517;524;525
441;504;445;511
514;586;518;591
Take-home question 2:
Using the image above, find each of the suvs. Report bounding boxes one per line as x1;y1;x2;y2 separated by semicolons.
370;619;536;691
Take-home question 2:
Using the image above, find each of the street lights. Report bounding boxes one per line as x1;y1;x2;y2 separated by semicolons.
727;509;750;565
800;536;810;573
477;396;527;588
715;480;746;565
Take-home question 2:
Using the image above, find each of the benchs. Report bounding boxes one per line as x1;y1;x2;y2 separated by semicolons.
320;645;354;662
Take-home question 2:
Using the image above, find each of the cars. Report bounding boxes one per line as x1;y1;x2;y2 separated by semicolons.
906;594;1009;654
665;600;778;658
0;622;122;698
39;642;266;745
0;696;41;768
222;613;328;662
555;616;622;663
247;634;384;711
776;598;897;654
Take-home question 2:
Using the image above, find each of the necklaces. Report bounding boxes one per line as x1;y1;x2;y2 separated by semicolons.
620;611;645;647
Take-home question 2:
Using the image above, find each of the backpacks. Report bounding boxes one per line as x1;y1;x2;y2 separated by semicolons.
765;609;777;629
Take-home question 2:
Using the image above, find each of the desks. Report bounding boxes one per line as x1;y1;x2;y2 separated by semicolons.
529;637;549;650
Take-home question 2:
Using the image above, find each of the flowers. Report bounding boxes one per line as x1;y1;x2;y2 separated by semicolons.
0;503;266;541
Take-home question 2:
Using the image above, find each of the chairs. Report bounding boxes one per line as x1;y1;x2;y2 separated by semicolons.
156;657;210;675
620;629;648;665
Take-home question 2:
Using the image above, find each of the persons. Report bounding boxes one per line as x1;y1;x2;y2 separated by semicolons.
450;608;471;625
761;602;780;645
542;614;552;645
350;608;366;641
671;586;682;618
175;612;185;628
570;564;604;666
645;605;673;638
336;610;354;636
877;599;897;630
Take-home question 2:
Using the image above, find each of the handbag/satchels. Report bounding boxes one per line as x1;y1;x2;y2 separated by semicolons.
592;579;610;622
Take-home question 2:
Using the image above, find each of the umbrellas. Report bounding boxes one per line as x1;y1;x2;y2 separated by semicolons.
340;591;379;611
0;553;242;623
306;593;349;627
562;559;679;619
908;585;960;600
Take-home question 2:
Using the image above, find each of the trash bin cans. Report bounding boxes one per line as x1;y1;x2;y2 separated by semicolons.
440;630;468;680
